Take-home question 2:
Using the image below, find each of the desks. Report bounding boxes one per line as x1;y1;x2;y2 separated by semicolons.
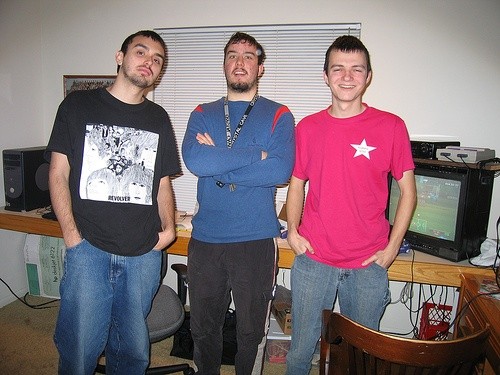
0;208;495;288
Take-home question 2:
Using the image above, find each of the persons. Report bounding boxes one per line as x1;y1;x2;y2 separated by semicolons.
286;35;417;375
45;30;186;375
181;32;293;375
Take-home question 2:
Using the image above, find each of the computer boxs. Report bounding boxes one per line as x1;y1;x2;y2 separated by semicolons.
2;146;51;212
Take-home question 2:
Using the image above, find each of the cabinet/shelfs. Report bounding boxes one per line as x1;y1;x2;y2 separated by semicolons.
453;273;500;375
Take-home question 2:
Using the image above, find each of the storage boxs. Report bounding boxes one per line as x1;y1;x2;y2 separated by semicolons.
24;234;67;299
266;316;291;363
271;296;292;335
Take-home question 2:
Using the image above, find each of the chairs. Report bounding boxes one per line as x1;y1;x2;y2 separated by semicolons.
170;259;244;366
320;309;490;375
100;250;188;375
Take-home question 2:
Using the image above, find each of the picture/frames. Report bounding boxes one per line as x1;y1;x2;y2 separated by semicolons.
63;75;117;99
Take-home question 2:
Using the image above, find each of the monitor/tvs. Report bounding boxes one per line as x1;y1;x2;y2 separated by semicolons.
384;163;495;263
